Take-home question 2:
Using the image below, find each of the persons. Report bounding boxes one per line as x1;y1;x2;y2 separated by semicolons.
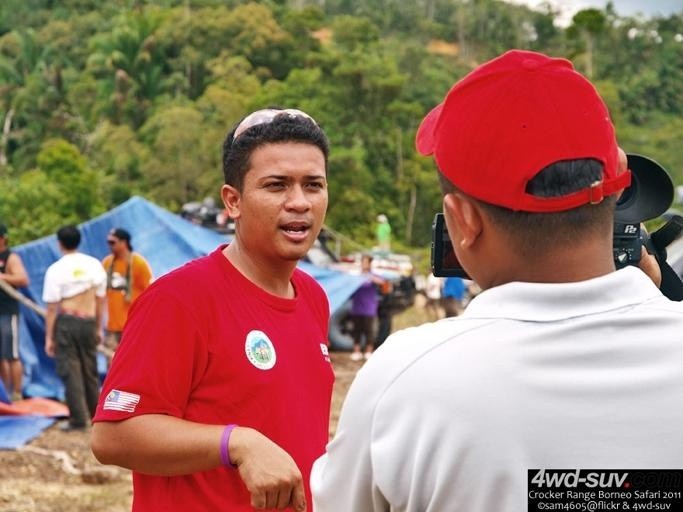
423;269;438;322
346;251;385;361
87;107;337;512
0;225;29;409
373;210;392;253
442;275;462;318
101;228;150;370
42;224;107;431
308;49;682;511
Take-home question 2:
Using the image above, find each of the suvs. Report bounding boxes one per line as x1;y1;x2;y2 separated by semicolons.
179;201;414;352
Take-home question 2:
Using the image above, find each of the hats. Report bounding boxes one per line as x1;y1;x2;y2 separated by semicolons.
108;227;133;252
417;48;631;213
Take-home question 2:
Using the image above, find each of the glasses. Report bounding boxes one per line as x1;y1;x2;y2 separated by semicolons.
109;241;116;244
231;109;320;148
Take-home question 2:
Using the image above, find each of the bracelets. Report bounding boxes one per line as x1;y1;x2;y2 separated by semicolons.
219;422;236;467
